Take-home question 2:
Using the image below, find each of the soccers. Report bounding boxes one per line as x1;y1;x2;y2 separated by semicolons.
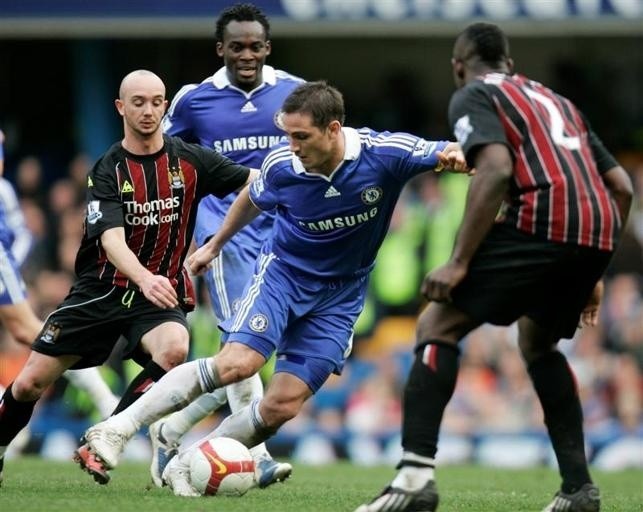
190;438;254;496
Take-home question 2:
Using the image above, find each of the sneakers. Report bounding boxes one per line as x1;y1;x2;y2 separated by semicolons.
542;483;600;512
73;419;293;499
352;479;438;510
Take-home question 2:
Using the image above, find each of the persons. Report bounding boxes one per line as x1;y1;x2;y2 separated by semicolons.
0;70;261;484
1;152;635;430
0;131;119;420
149;7;314;488
76;80;475;497
345;21;633;511
